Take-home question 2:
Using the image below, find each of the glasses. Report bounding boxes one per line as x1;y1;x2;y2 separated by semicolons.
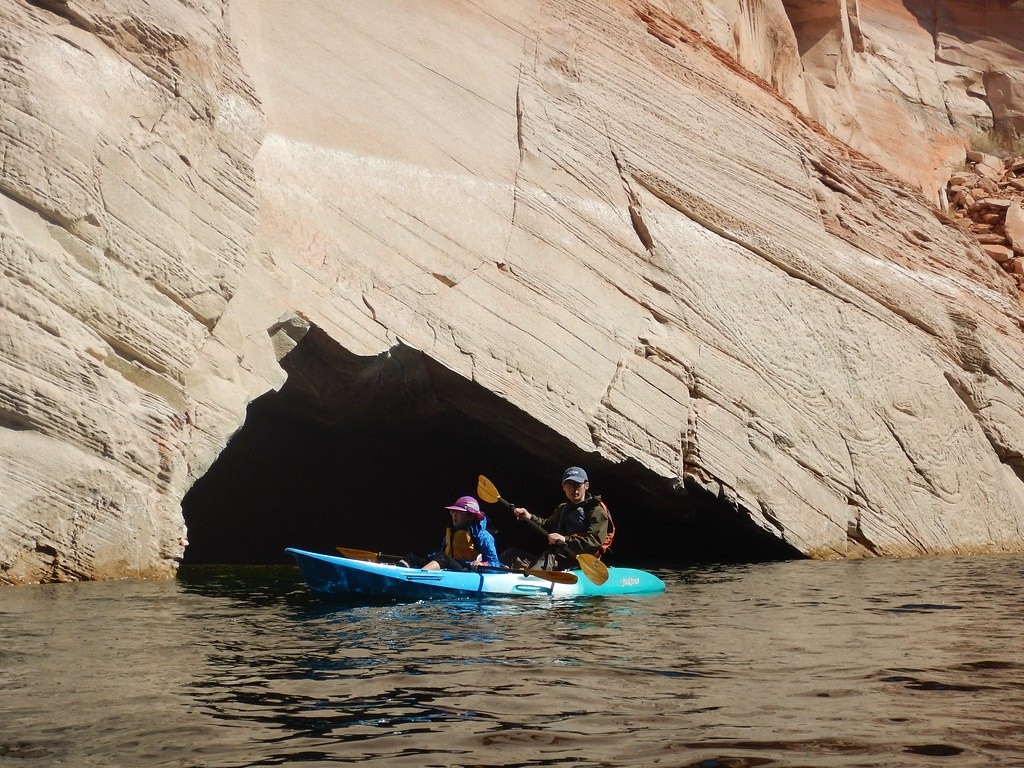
563;481;585;489
449;509;471;518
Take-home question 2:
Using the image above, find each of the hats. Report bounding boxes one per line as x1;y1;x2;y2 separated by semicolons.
560;466;589;486
444;496;486;521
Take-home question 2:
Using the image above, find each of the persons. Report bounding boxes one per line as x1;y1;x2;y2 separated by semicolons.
498;467;615;571
396;496;500;571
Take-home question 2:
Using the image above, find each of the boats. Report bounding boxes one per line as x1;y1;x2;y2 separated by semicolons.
285;547;668;605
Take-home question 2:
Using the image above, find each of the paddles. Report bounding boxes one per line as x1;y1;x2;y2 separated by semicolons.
336;546;579;584
477;474;610;587
